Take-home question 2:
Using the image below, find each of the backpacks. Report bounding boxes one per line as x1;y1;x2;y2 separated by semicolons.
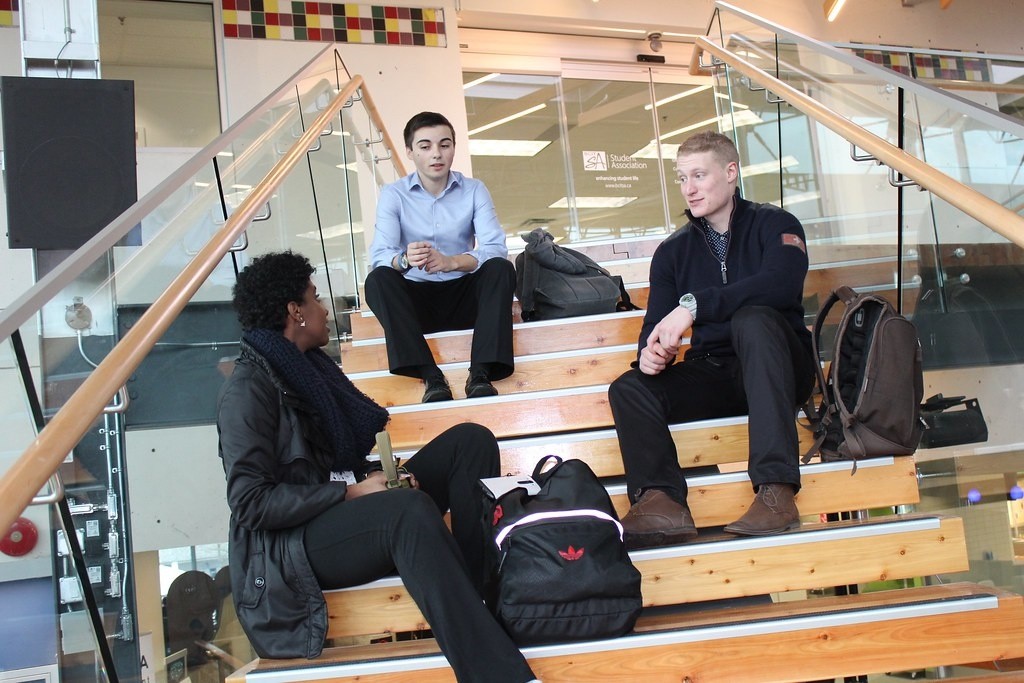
908;282;1017;370
516;246;641;321
471;453;643;647
796;280;927;475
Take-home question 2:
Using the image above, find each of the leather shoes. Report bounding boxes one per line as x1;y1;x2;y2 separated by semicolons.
464;370;498;399
422;374;452;404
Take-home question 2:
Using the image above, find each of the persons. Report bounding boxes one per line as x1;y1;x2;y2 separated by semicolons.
362;110;519;405
606;129;818;551
215;247;543;683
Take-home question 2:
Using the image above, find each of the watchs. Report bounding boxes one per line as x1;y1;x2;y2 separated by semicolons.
678;291;700;322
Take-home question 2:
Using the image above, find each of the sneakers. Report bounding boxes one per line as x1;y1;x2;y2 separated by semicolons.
619;487;698;550
723;482;799;536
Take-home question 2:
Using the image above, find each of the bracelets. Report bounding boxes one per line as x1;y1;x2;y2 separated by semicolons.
397;249;414;272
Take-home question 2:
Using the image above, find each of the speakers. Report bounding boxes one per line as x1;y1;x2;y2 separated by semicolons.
1;76;143;249
